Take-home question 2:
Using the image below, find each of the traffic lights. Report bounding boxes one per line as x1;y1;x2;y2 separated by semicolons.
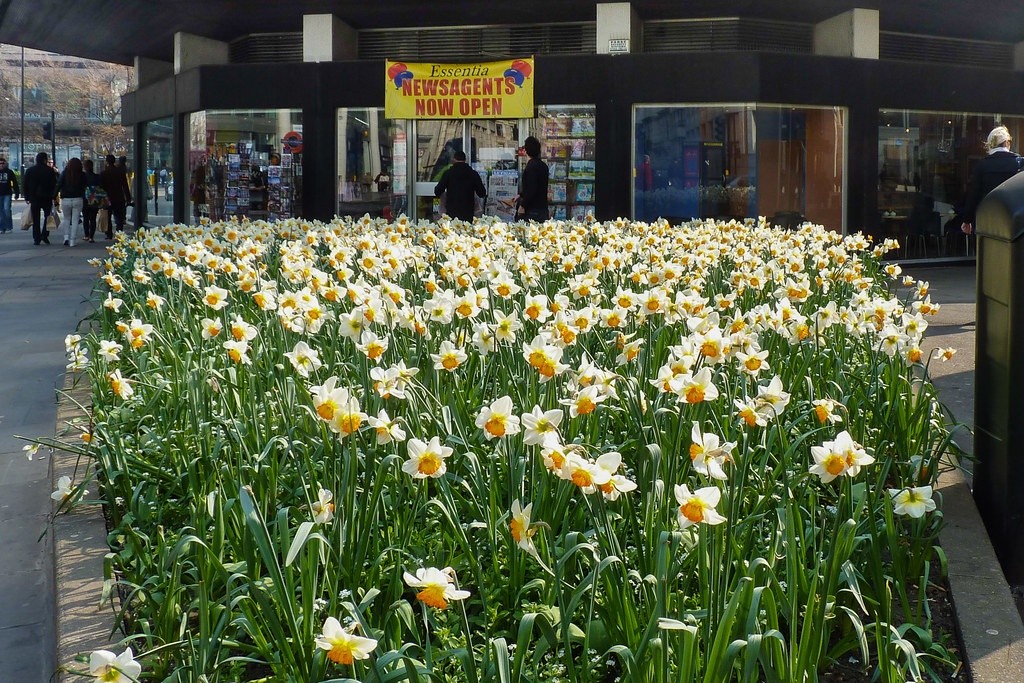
42;122;51;140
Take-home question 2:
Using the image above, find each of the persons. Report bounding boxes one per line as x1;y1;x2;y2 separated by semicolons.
54;154;136;247
373;167;391;194
23;153;61;246
960;125;1024;234
637;155;653;191
512;136;550;224
434;150;486;224
0;158;19;234
160;168;168;187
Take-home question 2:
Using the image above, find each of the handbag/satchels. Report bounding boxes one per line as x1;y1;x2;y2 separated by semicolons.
46;212;57;232
54;210;64;228
96;209;108;232
18;203;34;230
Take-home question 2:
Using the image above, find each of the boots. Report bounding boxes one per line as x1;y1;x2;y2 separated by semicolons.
63;235;69;245
70;240;78;247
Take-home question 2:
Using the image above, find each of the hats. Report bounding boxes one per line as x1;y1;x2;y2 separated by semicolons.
987;127;1012;147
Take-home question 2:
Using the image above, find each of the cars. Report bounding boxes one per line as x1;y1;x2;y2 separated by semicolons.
164;177;174;200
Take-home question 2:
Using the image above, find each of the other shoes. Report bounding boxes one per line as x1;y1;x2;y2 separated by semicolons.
5;230;12;234
33;235;43;245
82;237;89;240
0;231;4;234
89;239;95;243
105;237;112;240
41;236;50;244
